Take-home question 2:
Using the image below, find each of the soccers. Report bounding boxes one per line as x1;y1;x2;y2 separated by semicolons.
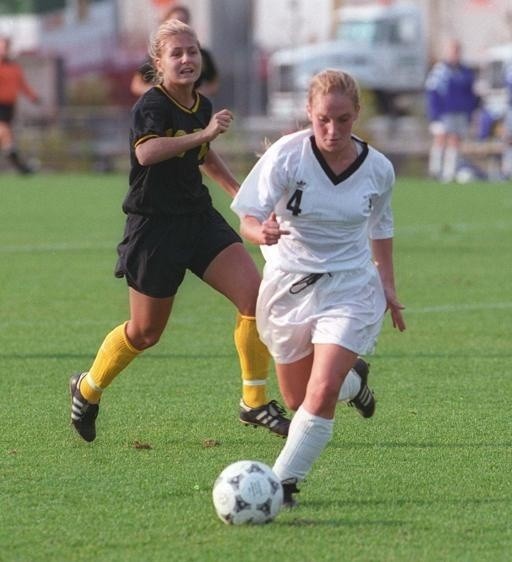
454;168;474;185
210;458;284;528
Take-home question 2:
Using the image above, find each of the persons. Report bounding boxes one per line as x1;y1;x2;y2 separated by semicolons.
67;19;292;444
226;67;410;512
422;35;480;186
0;31;44;179
454;61;511;184
128;4;221;104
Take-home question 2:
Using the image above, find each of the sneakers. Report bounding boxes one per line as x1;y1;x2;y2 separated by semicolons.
349;358;375;418
70;373;100;442
237;399;290;438
281;477;300;506
